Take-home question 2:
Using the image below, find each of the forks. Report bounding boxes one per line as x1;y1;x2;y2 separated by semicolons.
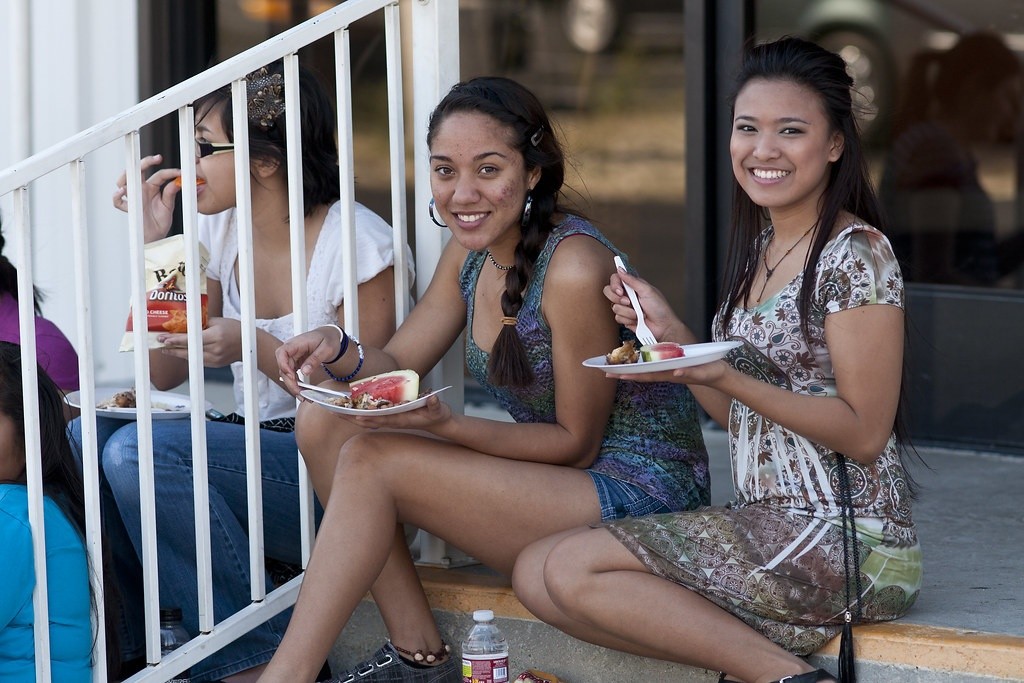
614;256;657;346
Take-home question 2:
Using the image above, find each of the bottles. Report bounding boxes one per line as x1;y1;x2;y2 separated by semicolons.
461;610;510;683
158;608;194;683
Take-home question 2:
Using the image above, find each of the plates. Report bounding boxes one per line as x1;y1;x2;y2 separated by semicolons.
64;388;212;420
582;342;745;373
300;386;452;417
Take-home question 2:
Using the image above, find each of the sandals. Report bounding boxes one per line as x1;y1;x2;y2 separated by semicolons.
717;668;846;683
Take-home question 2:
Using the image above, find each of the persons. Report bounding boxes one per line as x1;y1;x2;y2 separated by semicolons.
257;77;711;682
0;220;81;425
0;342;118;683
878;30;1021;287
513;37;923;683
65;60;415;683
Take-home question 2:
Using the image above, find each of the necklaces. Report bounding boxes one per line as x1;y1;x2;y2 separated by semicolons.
488;252;514;272
757;224;814;301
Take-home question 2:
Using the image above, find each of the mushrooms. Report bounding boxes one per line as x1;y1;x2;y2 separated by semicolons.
605;339;640;364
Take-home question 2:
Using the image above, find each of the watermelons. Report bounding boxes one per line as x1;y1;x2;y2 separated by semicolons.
349;369;420;404
640;341;685;362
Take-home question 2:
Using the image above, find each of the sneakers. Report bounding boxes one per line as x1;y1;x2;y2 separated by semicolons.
319;636;461;683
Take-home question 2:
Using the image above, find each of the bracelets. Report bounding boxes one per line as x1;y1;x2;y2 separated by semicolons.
323;336;364;381
322;324;349;364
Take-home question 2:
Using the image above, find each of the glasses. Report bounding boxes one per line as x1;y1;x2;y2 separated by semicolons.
194;137;233;159
